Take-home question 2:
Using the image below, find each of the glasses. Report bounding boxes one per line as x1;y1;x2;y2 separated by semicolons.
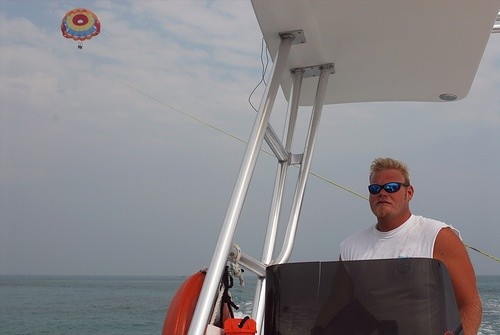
368;182;409;195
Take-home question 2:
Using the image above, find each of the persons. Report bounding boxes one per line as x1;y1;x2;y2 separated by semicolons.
340;157;483;335
